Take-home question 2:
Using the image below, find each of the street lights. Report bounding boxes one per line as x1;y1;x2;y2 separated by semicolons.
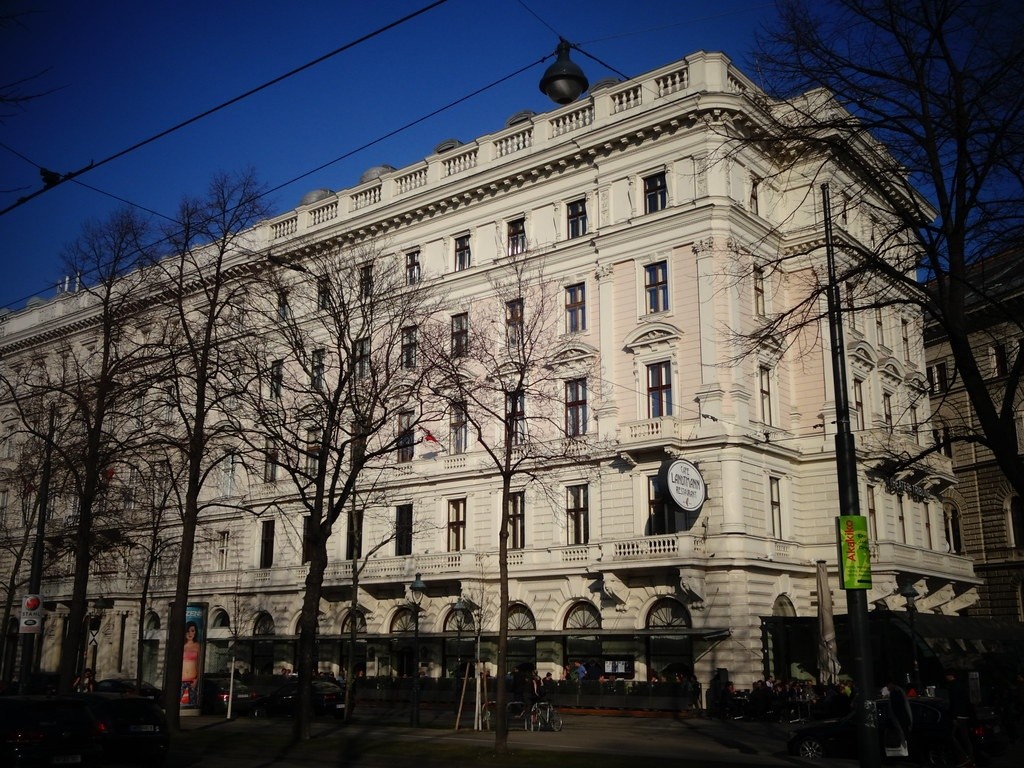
900;584;923;696
410;572;426;729
452;598;467;716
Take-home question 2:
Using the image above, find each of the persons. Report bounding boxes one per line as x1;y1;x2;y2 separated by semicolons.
219;662;917;723
179;621;201;709
943;674;976;768
875;669;912;768
73;668;94;694
1013;673;1024;742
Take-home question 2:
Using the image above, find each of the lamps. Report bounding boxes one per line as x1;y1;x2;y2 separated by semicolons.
538;41;589;105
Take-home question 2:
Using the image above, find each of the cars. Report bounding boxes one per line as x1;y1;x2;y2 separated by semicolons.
202;677;263;715
0;678;170;768
786;696;1008;768
268;680;354;718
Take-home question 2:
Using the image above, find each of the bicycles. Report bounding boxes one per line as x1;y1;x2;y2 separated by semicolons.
526;698;563;733
